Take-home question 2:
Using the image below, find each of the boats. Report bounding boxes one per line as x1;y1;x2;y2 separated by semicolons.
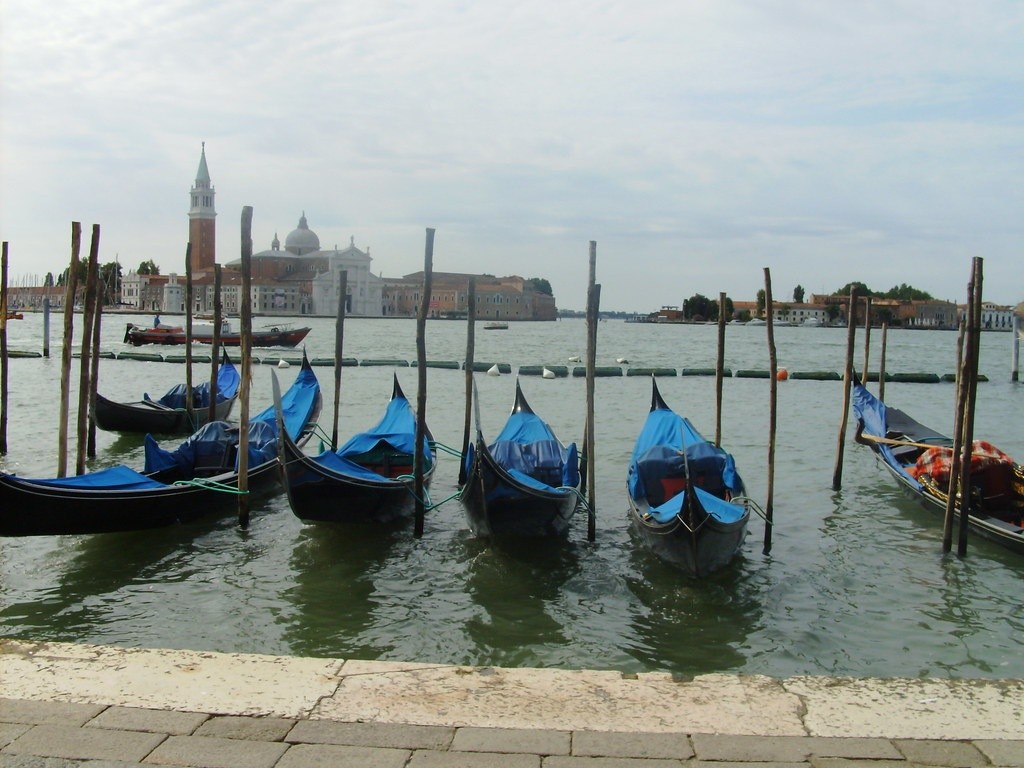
454;374;585;547
625;371;751;575
6;312;24;321
850;367;1024;554
268;364;440;522
126;318;312;350
95;344;240;434
729;318;792;327
0;344;324;536
486;321;509;330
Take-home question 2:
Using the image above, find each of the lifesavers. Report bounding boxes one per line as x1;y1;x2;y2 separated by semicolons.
133;338;144;346
165;336;175;344
256;337;266;346
279;333;287;346
